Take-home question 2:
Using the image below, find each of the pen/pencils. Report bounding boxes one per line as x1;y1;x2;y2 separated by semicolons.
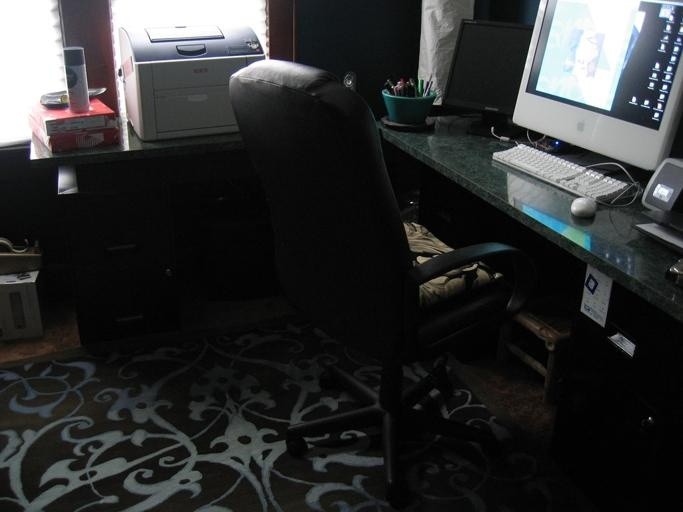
386;74;434;97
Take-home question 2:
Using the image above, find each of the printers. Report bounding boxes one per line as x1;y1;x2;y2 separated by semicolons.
118;21;265;143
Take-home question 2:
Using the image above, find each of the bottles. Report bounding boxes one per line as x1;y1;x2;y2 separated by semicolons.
63;46;90;114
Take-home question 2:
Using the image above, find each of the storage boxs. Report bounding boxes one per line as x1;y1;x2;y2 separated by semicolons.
0;268;47;343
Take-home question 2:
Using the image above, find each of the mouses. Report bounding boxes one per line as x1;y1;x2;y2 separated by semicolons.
570;197;598;217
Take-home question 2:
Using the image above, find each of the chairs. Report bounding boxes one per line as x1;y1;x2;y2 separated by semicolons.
229;58;528;485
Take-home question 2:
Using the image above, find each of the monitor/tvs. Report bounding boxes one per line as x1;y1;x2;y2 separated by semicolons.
512;1;683;175
442;17;535;140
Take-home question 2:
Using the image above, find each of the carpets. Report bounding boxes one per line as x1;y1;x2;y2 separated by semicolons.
1;314;562;512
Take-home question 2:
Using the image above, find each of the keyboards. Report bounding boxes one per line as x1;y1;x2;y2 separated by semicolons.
492;144;629;203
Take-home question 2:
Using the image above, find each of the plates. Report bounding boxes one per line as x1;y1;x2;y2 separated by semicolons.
381;116;435;130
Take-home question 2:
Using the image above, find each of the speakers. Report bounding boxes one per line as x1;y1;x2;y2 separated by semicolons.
640;156;683;223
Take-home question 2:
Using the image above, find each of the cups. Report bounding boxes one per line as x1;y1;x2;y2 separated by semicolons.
381;89;436;124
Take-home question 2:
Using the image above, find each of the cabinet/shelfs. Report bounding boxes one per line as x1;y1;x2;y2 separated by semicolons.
27;127;302;356
383;115;683;512
